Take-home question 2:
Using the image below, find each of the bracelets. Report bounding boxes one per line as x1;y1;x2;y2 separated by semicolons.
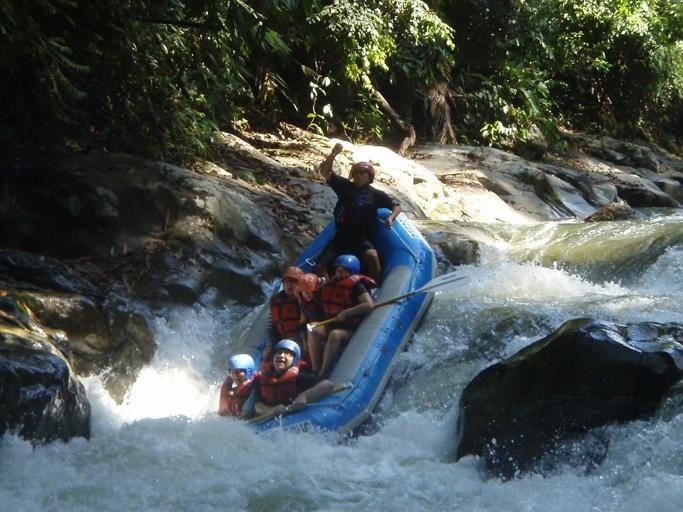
329;152;336;160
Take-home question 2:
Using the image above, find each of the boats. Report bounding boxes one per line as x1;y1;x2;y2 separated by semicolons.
230;208;438;446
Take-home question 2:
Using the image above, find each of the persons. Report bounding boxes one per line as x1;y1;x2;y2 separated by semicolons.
251;266;304;370
219;353;257;415
305;254;375;379
290;273;327;360
239;339;333;419
313;143;401;287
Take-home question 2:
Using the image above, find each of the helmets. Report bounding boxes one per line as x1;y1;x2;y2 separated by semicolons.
270;339;301;366
296;273;321;293
282;267;304;283
334;255;361;275
348;162;375;184
225;354;255;380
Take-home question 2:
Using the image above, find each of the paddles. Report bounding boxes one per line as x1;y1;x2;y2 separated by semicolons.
306;270;472;333
